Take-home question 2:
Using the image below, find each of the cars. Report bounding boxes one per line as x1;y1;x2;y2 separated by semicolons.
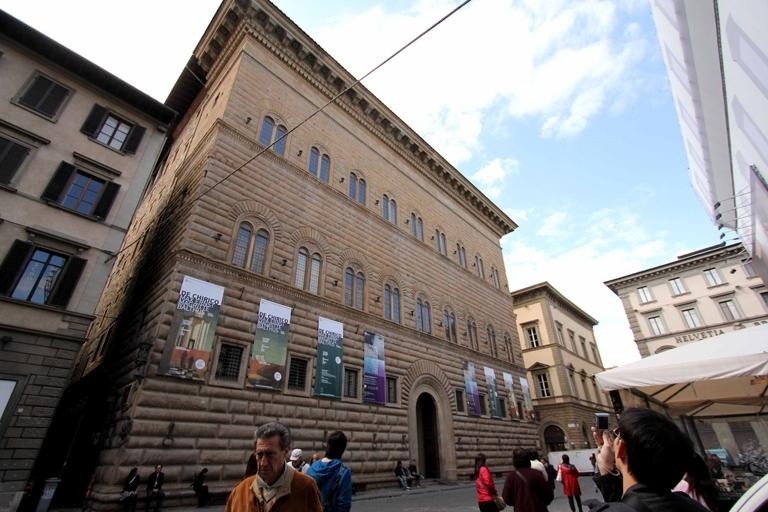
705;448;734;465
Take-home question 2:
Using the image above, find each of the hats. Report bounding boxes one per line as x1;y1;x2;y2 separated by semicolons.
289;449;303;461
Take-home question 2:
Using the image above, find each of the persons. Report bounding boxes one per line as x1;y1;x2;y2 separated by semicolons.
394;459;425;491
118;463;165;510
224;422;351;512
472;408;723;511
192;467;210;508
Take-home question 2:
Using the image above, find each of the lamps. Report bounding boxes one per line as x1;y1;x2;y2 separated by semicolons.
713;184;753;275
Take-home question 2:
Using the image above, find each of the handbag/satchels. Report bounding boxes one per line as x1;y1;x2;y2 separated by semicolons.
494;496;507;511
555;469;563;482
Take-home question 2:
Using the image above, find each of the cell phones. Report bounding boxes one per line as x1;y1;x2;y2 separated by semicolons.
595;412;610;435
